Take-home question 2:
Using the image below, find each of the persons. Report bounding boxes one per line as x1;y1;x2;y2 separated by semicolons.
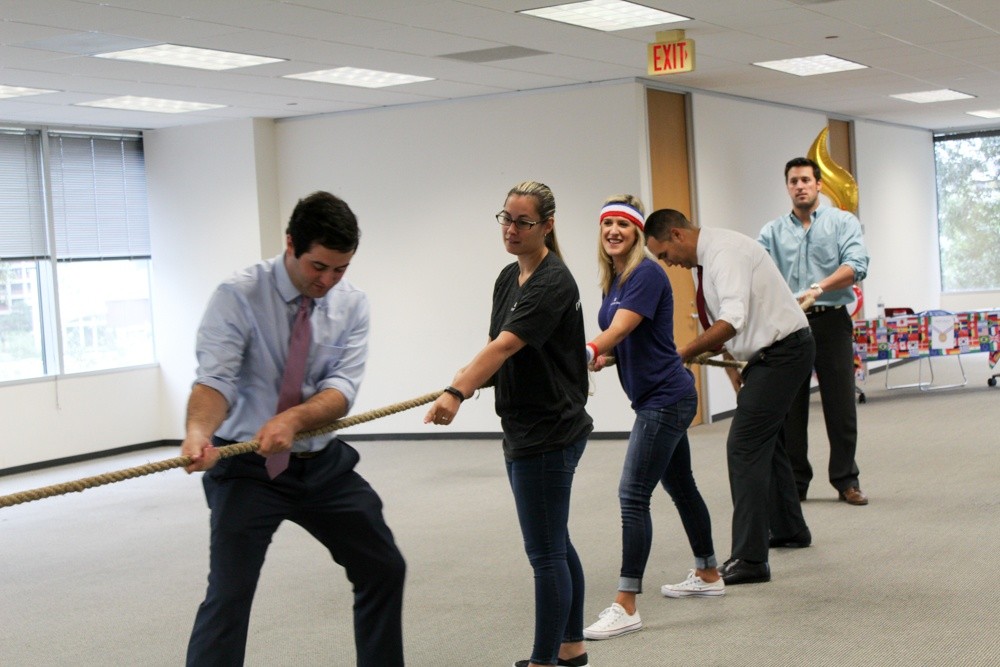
423;189;593;667
582;195;726;640
644;206;816;584
180;191;405;667
754;157;869;506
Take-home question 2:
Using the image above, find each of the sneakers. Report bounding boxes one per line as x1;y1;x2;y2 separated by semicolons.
581;603;642;640
661;569;725;599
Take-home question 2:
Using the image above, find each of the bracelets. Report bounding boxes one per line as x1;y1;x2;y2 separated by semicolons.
443;387;464;403
587;342;599;362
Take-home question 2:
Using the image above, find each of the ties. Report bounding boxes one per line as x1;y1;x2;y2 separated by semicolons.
694;266;711;331
264;294;314;479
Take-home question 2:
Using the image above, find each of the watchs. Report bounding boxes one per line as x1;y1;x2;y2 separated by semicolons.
810;282;823;296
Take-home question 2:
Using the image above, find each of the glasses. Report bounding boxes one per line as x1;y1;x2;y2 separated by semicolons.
495;210;541;232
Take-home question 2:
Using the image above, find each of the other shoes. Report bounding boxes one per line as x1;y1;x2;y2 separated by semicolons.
514;652;591;667
838;488;869;505
717;558;771;584
767;530;812;548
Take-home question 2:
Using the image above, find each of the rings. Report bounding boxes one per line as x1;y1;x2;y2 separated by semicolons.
441;416;448;420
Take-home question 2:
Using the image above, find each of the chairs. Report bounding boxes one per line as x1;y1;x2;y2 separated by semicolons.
888;309;969;392
